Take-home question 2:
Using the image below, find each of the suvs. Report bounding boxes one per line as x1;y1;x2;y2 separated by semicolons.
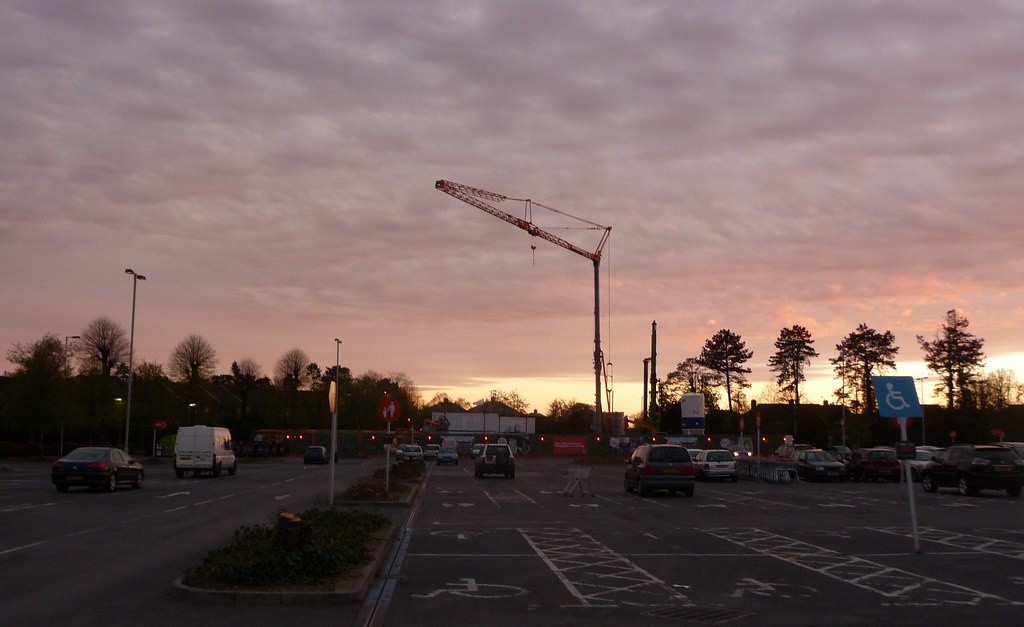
922;444;1024;496
474;443;515;479
624;443;694;498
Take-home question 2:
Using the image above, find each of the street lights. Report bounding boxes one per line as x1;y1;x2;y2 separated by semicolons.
125;269;146;453
65;336;81;377
331;338;342;509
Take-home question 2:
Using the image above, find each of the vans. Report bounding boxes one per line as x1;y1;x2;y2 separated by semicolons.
175;426;237;478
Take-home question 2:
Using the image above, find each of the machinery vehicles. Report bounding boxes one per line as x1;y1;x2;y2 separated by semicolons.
436;179;631;462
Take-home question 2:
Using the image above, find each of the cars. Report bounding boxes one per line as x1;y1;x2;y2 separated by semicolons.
397;444;407;459
425;444;441;459
52;448;145;492
688;449;704;472
402;445;424;462
305;446;329;465
471;444;485;459
436;447;459;466
693;450;739;483
774;444;946;483
